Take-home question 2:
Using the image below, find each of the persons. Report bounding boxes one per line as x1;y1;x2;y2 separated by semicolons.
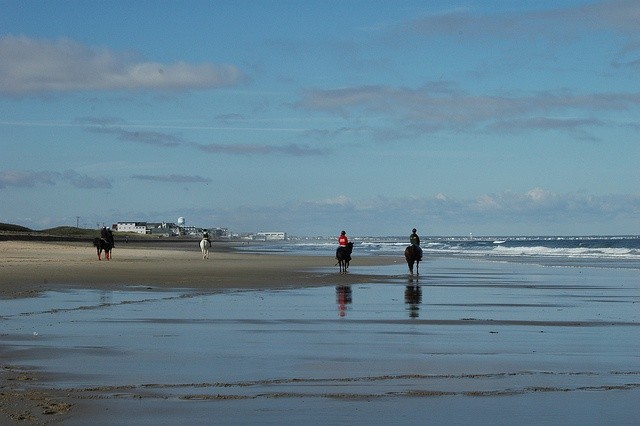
409;228;422;261
336;231;351;258
199;229;212;247
101;227;107;238
106;227;115;248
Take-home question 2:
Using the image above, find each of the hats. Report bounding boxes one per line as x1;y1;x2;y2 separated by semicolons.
413;228;416;232
341;231;346;233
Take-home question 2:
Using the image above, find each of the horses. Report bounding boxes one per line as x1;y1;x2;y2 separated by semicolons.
199;239;211;260
334;241;354;273
93;235;112;261
405;245;423;278
